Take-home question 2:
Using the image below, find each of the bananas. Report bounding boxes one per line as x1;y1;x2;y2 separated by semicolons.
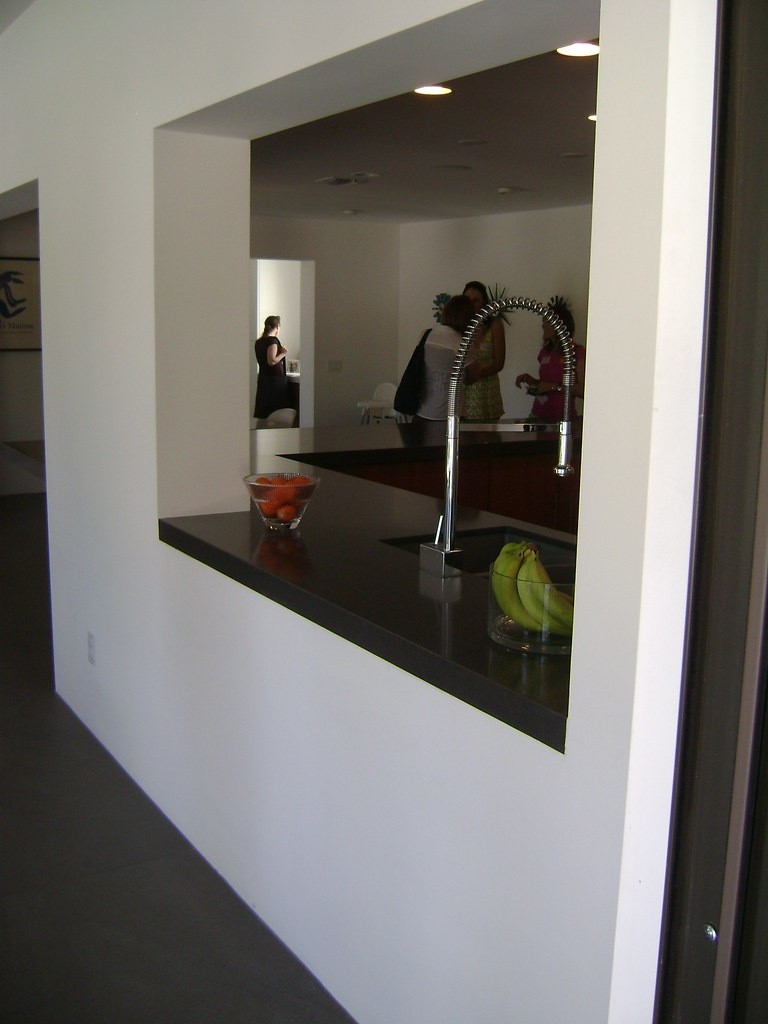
491;540;574;636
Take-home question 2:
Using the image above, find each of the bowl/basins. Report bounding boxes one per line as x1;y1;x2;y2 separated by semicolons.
488;561;576;654
244;473;321;529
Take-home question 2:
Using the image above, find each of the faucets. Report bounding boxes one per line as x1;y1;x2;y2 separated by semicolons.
419;296;575;578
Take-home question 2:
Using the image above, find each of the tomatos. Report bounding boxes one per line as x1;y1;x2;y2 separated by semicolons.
252;475;312;521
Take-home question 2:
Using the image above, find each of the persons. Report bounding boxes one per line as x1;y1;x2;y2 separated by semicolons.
253;316;288;420
411;295;479;423
515;305;585;421
455;280;507;420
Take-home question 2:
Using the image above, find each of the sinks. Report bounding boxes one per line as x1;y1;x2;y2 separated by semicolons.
378;525;578;581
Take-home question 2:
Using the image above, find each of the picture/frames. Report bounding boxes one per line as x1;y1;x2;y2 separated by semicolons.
0;256;42;351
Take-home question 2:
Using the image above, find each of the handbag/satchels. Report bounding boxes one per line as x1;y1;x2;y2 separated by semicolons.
393;327;433;417
